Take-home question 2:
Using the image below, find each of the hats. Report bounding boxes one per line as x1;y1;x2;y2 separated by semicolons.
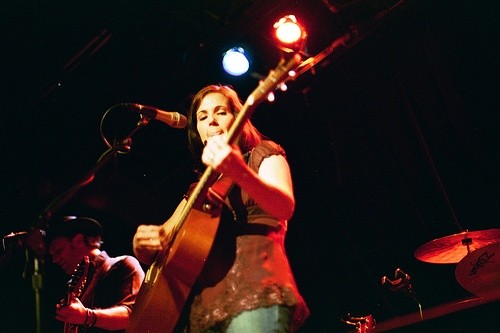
43;215;102;249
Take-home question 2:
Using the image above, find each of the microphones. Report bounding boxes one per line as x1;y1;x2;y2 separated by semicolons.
3;230;46;239
122;102;187;128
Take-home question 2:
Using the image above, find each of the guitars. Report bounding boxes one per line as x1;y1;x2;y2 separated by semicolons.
128;46;305;333
59;254;93;333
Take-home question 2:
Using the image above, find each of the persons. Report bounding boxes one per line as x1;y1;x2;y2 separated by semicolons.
132;85;309;333
44;216;146;333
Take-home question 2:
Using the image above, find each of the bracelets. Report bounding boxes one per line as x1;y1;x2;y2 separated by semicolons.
85;308;97;327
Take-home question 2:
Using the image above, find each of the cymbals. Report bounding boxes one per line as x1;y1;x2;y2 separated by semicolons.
455;241;500;300
414;229;500;264
